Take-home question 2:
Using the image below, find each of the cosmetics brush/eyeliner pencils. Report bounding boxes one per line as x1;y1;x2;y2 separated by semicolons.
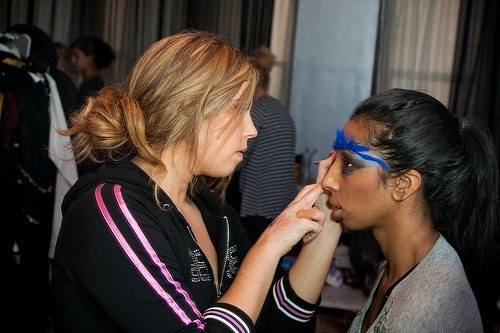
313;159;320;164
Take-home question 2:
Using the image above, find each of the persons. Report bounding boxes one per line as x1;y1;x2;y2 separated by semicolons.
321;86;500;332
71;35;114;112
293;151;384;297
44;29;343;333
49;42;81;126
213;44;297;246
0;23;82;333
